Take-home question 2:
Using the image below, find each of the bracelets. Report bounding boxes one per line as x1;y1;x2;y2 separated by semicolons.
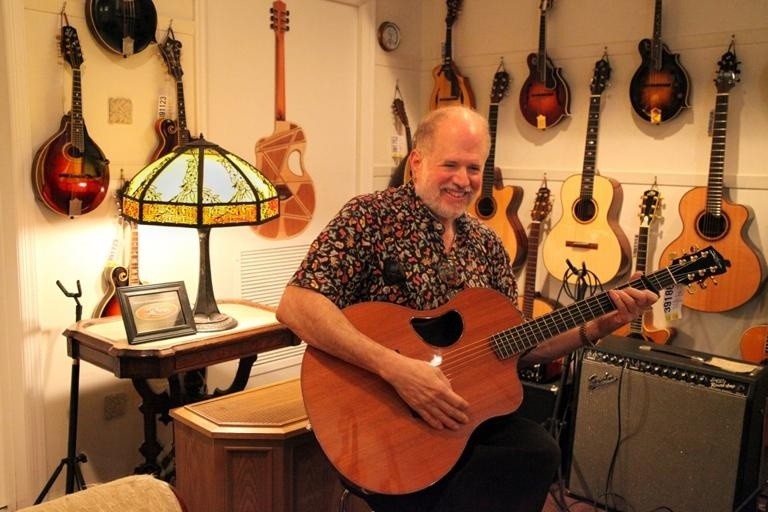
578;321;590;348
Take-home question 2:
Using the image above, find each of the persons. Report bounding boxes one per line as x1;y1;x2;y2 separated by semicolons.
275;106;659;511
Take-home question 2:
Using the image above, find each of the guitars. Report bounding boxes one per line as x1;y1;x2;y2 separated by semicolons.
516;1;573;131
658;52;768;311
32;26;109;216
740;325;768;366
301;246;726;495
95;180;153;318
85;0;157;56
152;37;202;162
387;96;414;191
606;187;678;345
517;186;564;322
426;0;477;111
542;58;632;286
248;2;316;239
628;0;695;124
463;62;529;273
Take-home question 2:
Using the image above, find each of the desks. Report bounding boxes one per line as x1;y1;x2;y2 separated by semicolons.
63;299;302;486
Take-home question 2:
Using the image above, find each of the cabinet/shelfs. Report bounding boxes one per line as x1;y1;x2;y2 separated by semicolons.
168;378;371;512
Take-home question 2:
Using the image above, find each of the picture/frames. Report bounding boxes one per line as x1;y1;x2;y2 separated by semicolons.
116;280;198;344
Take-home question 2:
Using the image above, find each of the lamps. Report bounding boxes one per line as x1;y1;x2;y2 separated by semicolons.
120;133;281;333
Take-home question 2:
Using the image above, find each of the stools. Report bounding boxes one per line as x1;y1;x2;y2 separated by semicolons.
338;478;371;511
